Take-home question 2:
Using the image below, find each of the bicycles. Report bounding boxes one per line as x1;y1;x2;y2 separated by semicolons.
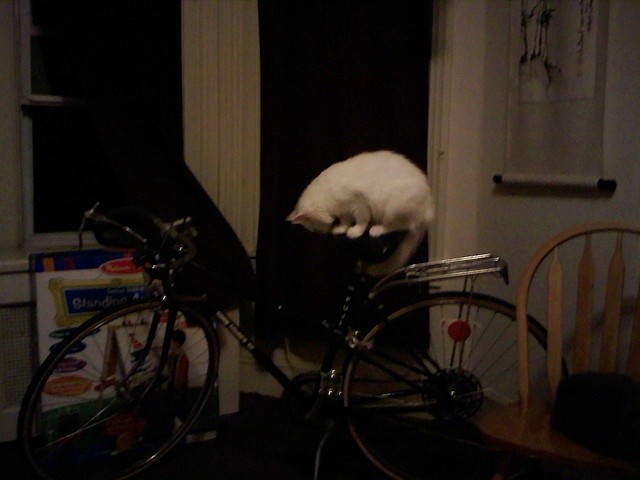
17;202;568;480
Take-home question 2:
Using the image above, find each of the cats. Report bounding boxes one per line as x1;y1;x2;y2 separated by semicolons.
287;149;436;278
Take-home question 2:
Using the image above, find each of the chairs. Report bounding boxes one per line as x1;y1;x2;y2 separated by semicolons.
476;224;639;473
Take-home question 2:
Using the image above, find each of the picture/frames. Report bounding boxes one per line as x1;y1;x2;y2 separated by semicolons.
488;1;618;196
